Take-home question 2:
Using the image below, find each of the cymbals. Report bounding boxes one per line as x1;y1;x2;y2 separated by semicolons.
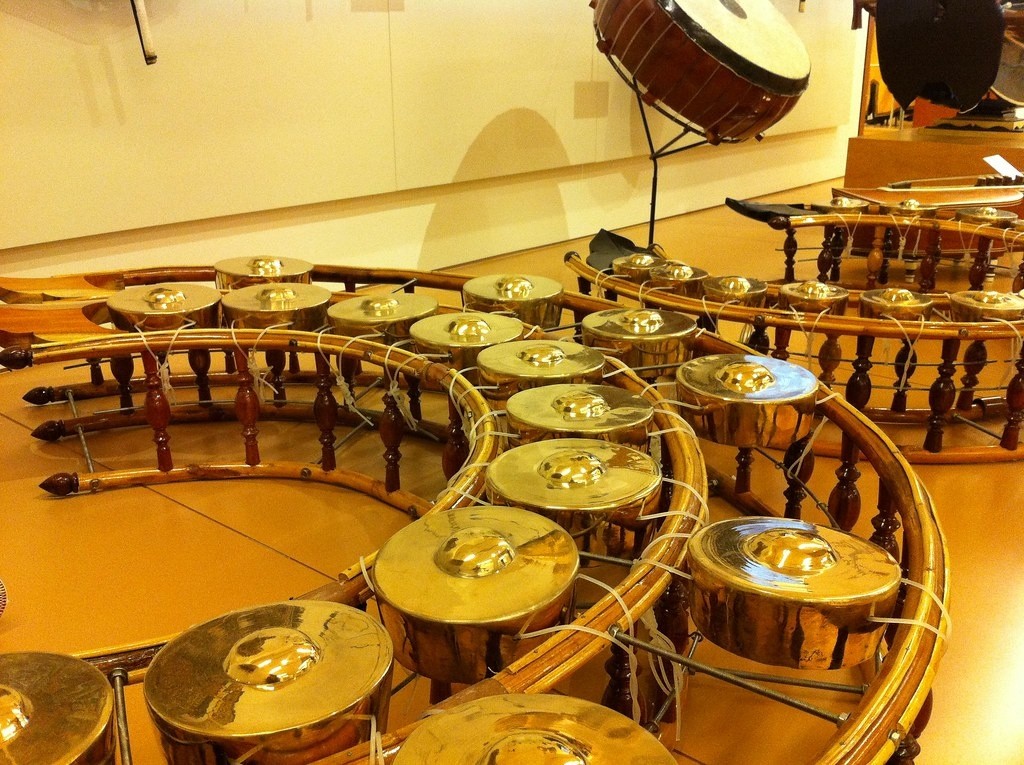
0;253;904;764
608;191;1024;328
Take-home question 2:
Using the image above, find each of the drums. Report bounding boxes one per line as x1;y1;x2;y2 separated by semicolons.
589;0;811;144
